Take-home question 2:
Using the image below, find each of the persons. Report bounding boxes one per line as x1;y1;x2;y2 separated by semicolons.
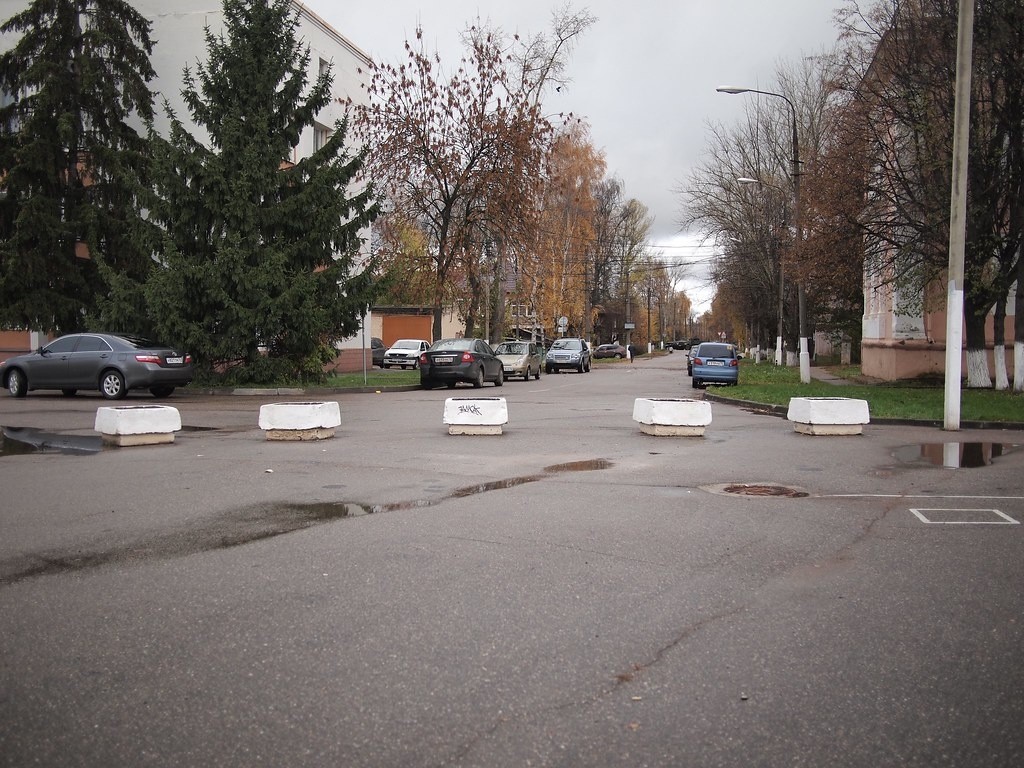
628;342;636;363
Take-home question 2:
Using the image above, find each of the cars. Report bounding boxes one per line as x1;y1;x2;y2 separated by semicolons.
0;332;195;399
418;338;505;388
690;342;742;386
494;341;542;380
664;340;700;350
370;338;386;369
382;339;431;370
593;343;626;360
685;345;700;377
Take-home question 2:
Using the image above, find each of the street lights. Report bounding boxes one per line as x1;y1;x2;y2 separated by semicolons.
716;85;811;385
737;177;787;367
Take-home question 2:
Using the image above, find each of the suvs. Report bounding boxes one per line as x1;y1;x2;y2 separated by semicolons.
544;338;592;373
